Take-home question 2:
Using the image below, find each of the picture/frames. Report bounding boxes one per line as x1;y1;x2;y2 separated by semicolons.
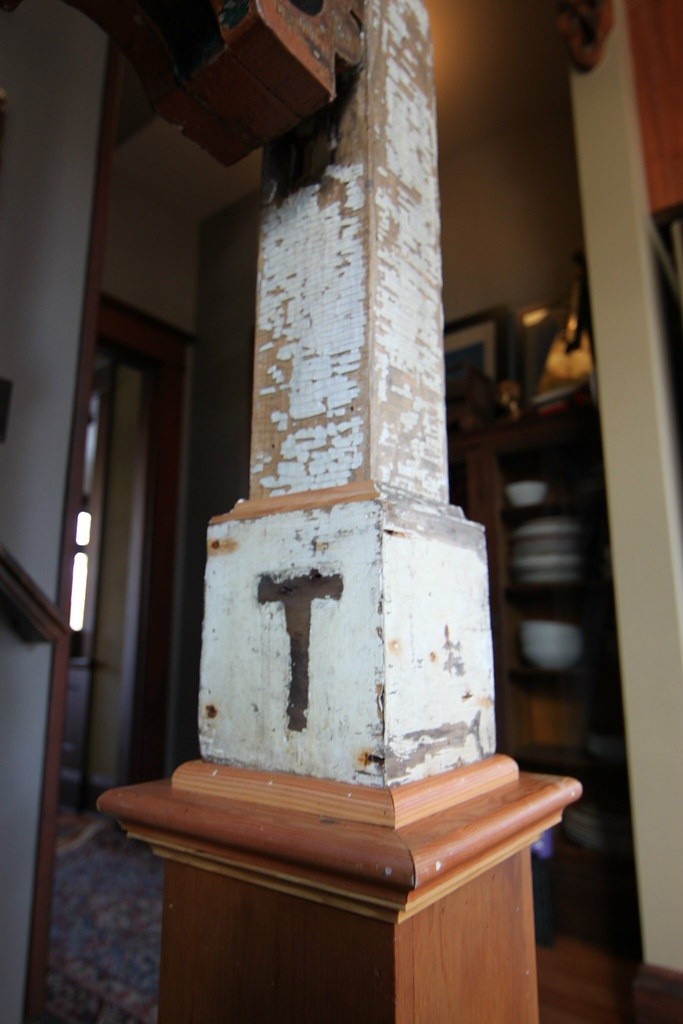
518;300;577;408
444;309;511;421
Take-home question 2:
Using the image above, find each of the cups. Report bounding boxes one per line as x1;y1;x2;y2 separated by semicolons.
521;620;582;670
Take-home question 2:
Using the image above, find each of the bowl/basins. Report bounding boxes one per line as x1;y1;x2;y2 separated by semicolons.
506;480;547;505
510;517;581;585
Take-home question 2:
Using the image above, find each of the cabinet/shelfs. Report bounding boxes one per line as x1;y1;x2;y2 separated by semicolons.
455;413;642;963
